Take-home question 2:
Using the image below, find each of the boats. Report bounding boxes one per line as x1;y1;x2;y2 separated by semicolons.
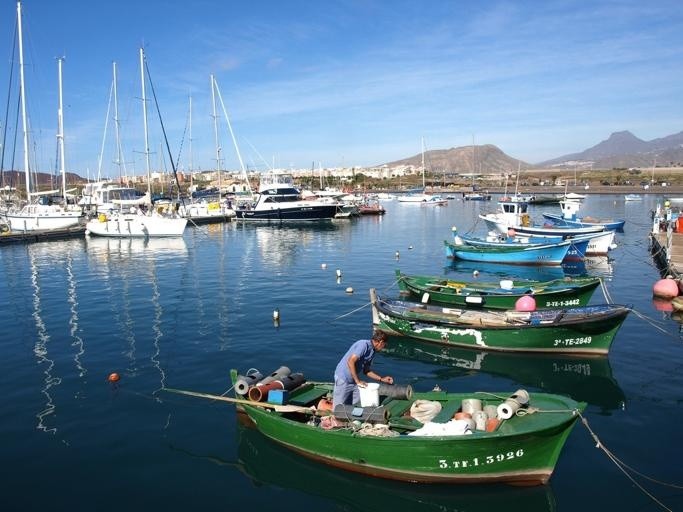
394;268;601;309
366;286;633;356
652;193;682;234
227;368;587;487
234;421;558;512
441;163;625;267
623;191;645;203
376;333;627;417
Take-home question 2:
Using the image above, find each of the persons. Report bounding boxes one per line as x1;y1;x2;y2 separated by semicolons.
333;330;393;413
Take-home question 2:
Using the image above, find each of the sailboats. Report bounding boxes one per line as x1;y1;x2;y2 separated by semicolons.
396;135;447;206
